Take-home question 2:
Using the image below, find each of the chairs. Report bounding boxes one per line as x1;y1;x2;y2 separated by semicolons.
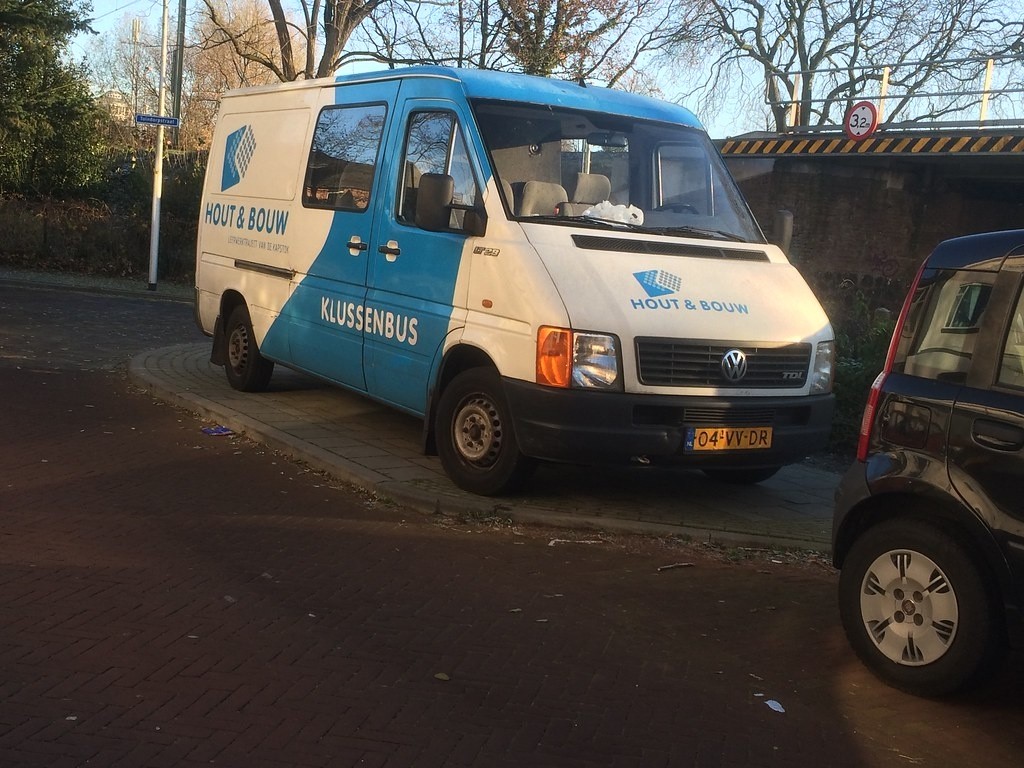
331;157;613;236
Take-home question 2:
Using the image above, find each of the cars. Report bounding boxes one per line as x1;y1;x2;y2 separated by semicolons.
826;230;1024;703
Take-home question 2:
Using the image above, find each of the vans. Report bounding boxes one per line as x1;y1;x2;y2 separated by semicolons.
193;65;835;500
